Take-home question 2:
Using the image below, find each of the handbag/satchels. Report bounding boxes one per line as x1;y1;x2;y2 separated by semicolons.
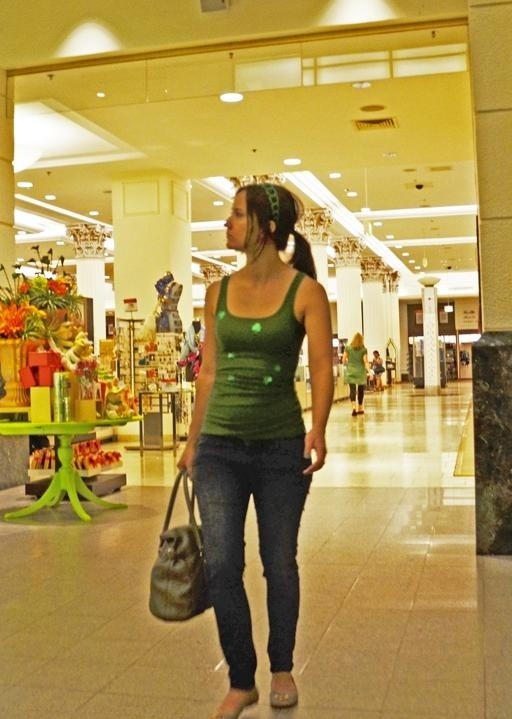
149;466;213;621
374;362;385;375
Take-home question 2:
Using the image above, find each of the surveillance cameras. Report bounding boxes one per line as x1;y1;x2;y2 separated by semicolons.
447;265;453;271
412;182;426;191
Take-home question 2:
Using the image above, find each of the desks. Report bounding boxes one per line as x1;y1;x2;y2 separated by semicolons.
0;416;142;522
138;390;195;457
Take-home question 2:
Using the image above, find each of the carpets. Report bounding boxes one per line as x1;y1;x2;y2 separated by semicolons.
453;399;476;476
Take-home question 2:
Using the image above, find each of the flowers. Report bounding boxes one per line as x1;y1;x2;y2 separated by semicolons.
0;245;84;358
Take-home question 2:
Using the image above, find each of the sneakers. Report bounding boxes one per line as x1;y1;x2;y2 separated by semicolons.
270;674;298;708
209;685;259;719
352;408;358;416
358;411;364;414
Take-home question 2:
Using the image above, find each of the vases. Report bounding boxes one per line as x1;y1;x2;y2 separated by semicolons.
0;339;31;407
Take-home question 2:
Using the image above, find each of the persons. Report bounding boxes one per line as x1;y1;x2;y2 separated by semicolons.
155;273;185;334
173;180;339;719
175;318;208;380
342;331;374;416
372;349;384;391
366;361;376;391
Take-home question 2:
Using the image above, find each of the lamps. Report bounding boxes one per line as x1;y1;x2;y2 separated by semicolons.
362;167;372;237
422;228;428;268
444;299;454;313
